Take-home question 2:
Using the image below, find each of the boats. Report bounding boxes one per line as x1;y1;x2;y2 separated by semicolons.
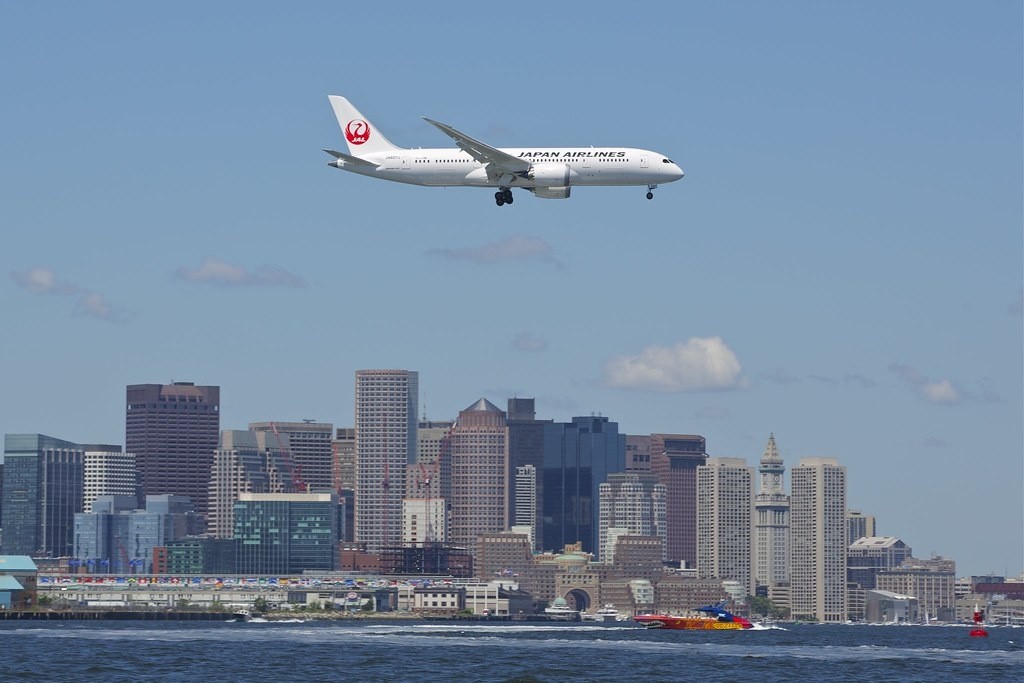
633;605;755;630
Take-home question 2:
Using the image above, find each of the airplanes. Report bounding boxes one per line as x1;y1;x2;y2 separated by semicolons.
322;94;685;206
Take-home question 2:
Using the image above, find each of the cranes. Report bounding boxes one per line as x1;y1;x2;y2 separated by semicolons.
416;439;449;548
271;422;305;491
